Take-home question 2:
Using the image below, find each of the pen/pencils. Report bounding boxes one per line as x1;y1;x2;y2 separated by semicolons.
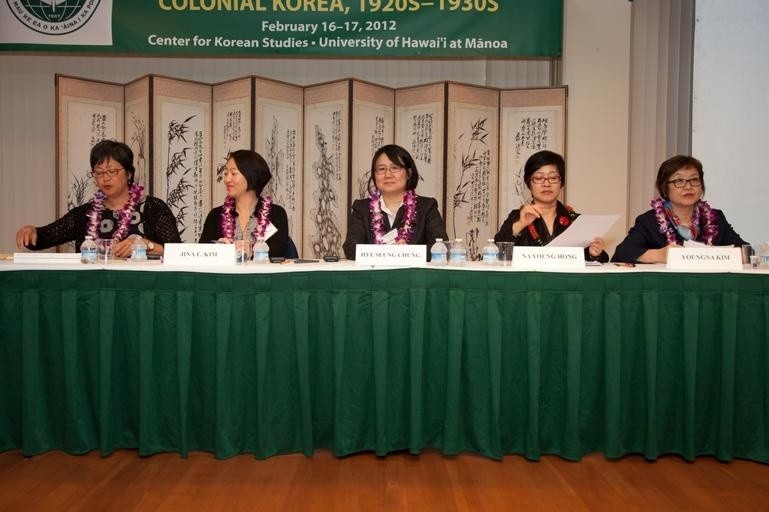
211;239;234;244
614;263;636;267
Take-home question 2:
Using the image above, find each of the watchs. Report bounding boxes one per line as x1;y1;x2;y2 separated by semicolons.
147;239;155;252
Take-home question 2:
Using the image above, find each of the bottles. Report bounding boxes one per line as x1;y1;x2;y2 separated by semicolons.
131;235;147;262
759;241;769;269
482;239;500;266
430;238;448;266
252;237;269;264
450;238;467;265
81;236;98;263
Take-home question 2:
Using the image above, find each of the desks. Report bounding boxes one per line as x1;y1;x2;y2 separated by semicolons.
0;258;769;464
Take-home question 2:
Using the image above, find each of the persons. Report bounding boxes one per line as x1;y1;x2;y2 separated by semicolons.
198;150;289;260
611;154;755;266
494;150;609;263
15;141;182;259
342;144;451;262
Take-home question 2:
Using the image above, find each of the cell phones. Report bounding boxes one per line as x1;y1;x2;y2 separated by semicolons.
148;254;158;260
270;256;285;263
324;256;339;262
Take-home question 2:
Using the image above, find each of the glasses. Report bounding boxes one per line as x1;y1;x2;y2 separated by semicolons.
531;175;560;185
378;164;403;175
667;176;701;188
90;168;123;177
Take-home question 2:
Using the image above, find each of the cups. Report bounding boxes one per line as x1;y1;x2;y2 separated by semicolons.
496;241;515;267
233;241;251;266
97;239;116;262
741;245;759;271
443;241;454;262
